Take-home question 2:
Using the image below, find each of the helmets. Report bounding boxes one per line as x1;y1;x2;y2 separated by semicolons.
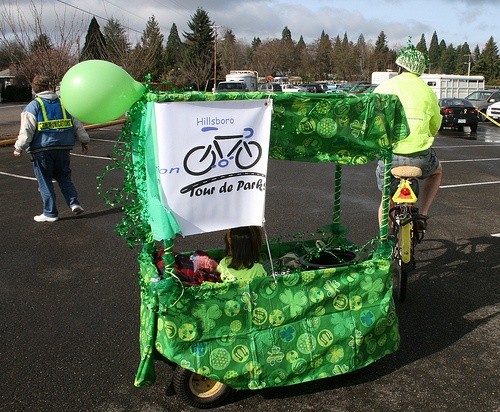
395;49;426;76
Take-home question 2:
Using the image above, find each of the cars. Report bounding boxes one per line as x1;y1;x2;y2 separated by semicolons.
485;100;500;122
215;69;380;95
437;97;479;132
461;88;500;122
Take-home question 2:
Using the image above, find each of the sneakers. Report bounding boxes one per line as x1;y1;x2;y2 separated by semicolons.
72;204;84;214
33;213;58;222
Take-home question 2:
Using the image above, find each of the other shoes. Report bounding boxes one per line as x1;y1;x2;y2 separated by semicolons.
417;214;429;229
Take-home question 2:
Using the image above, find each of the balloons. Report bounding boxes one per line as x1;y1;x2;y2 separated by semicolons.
59;59;146;125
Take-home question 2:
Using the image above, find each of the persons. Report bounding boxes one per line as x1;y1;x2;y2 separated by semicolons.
371;49;443;257
14;75;91;222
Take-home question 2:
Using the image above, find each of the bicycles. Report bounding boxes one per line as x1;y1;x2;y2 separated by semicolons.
387;176;426;304
